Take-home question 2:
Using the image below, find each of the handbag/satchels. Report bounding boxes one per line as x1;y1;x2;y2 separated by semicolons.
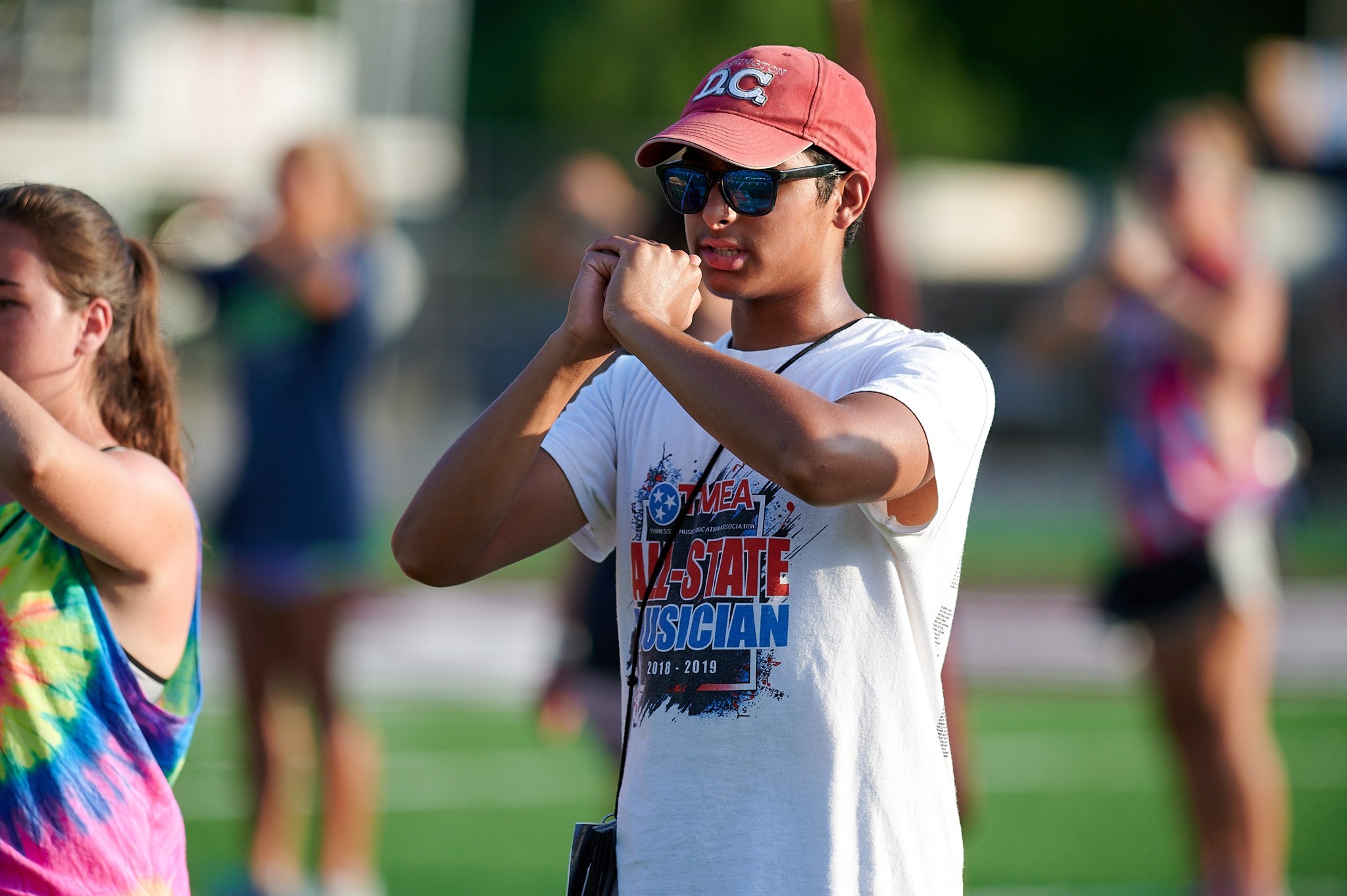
567;815;619;896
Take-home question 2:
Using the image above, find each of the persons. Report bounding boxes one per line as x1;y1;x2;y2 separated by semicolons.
0;182;204;896
390;44;996;896
146;142;645;896
1022;108;1295;896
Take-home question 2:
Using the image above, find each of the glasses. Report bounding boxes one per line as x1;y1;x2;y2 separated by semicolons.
653;158;844;219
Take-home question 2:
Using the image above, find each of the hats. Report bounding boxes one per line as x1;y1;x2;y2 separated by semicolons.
634;41;877;193
